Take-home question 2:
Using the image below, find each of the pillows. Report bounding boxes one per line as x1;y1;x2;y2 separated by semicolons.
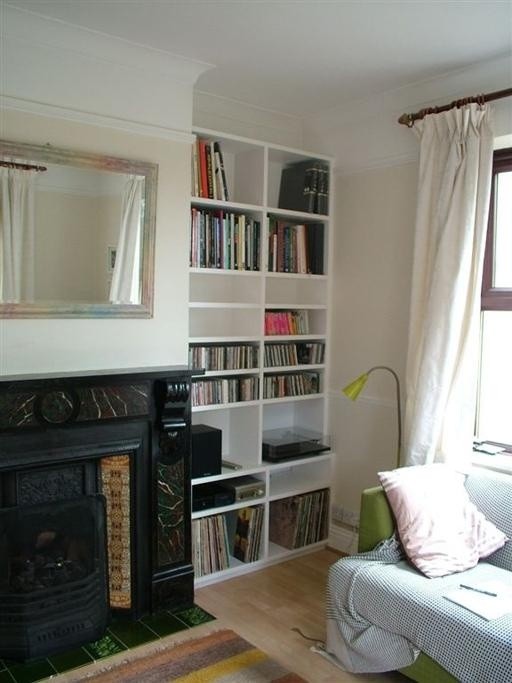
377;463;510;578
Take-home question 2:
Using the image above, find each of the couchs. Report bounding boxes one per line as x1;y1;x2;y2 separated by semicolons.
326;464;512;682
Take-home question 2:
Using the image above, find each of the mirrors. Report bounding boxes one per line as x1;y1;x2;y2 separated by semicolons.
0;140;160;317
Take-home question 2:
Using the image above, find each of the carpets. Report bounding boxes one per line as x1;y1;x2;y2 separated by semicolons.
72;627;309;683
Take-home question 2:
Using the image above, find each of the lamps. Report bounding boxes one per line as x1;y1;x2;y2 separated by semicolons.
343;366;401;468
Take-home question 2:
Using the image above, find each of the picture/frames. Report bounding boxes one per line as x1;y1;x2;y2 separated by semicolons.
106;245;119;273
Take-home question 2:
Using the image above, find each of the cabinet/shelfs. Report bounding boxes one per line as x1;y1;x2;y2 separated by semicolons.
190;123;335;591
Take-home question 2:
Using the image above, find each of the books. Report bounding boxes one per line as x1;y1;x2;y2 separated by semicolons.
190;135;327;274
192;486;328;575
188;309;326;407
444;579;512;621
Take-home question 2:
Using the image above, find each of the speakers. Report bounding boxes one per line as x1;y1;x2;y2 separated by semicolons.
191;424;222;479
